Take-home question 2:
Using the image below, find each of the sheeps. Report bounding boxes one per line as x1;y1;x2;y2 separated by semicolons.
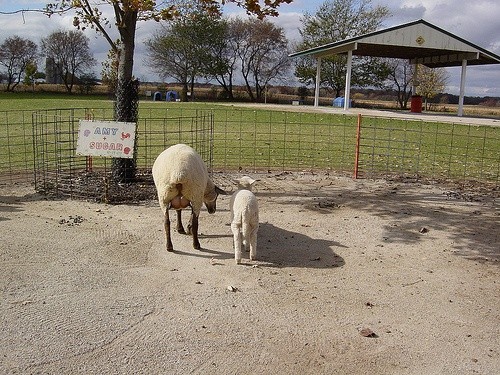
152;144;227;252
230;176;258;265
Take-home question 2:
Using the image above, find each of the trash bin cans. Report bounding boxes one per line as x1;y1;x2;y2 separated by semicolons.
333;96;351;108
166;91;178;102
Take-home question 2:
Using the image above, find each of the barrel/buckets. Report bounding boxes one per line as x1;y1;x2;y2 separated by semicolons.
411;95;422;113
